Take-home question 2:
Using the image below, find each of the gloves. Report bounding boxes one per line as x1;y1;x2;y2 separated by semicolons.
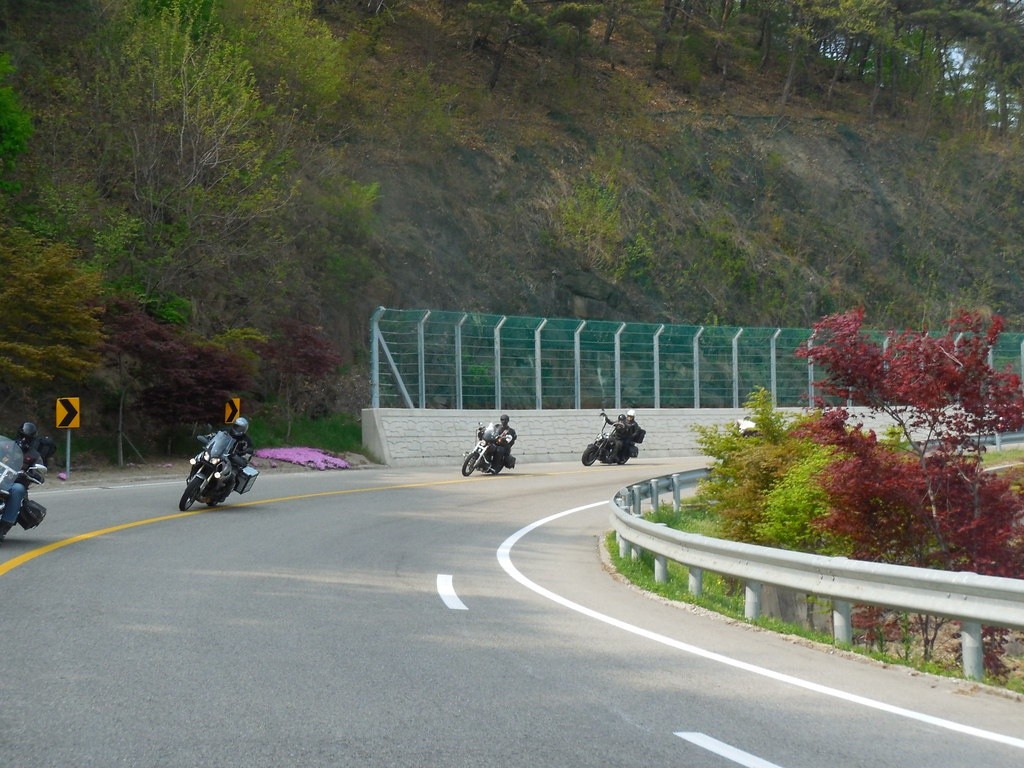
196;435;212;445
234;457;248;467
601;413;608;417
27;467;42;483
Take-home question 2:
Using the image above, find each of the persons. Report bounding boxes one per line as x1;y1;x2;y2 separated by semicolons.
203;417;254;496
0;421;45;534
600;410;638;461
480;415;517;469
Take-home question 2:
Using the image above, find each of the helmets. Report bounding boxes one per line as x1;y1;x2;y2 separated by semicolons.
626;408;636;419
231;417;250;436
500;414;509;421
16;422;39;452
618;414;626;423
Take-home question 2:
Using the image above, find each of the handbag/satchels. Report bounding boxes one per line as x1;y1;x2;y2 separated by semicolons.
17;497;47;531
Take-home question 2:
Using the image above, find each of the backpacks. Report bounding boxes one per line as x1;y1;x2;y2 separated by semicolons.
633;429;646;443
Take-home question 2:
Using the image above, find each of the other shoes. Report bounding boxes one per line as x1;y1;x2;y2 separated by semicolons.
216;496;226;502
607;456;626;463
490;467;498;473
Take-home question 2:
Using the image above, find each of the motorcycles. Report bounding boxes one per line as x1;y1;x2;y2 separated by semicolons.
179;422;261;512
462;421;517;476
582;407;646;466
0;433;59;540
